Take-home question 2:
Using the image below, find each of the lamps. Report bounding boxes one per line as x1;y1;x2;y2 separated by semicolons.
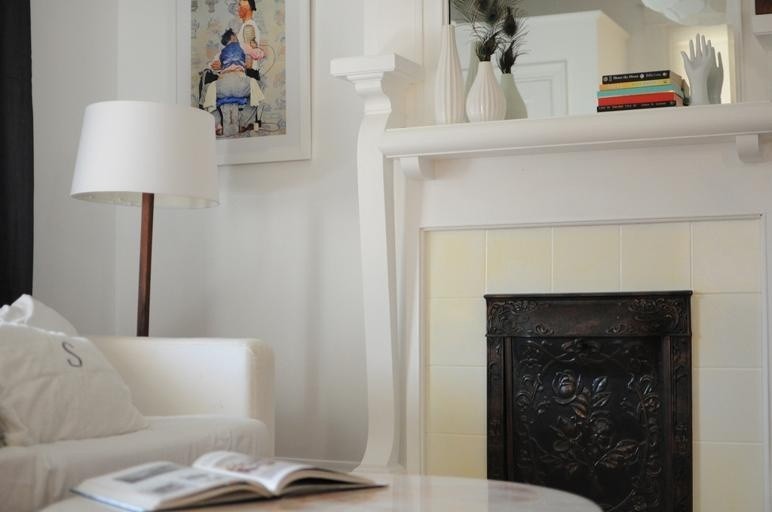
69;99;222;336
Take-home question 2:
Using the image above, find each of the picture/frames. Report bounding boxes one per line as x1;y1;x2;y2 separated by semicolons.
174;2;312;164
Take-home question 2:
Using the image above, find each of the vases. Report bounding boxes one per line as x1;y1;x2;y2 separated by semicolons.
436;25;508;123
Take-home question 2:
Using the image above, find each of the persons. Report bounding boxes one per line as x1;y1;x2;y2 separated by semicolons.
680;32;718;106
199;0;264;135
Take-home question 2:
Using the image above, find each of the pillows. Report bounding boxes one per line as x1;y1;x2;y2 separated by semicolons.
2;293;151;439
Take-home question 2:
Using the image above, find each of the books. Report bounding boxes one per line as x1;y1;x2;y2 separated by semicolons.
69;451;390;512
597;70;684;112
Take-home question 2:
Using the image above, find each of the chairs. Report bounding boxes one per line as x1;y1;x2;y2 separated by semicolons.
1;335;275;511
